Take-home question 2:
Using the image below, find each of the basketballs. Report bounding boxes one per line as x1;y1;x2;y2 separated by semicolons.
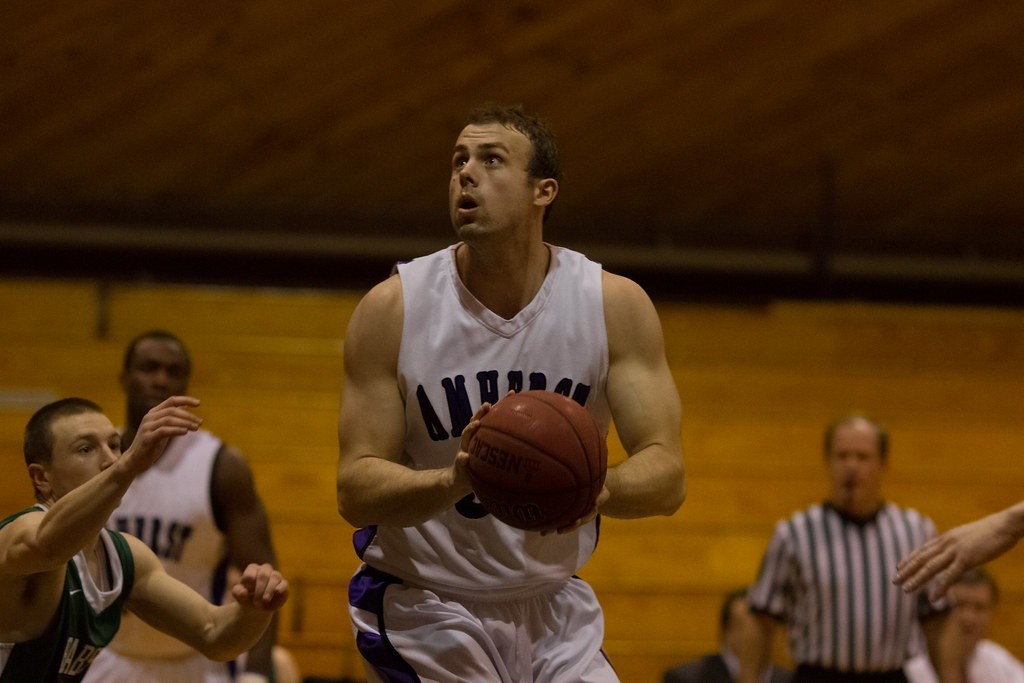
467;390;608;532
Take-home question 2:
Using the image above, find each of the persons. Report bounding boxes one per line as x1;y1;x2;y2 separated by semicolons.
337;105;687;683
662;416;1024;683
0;331;302;683
